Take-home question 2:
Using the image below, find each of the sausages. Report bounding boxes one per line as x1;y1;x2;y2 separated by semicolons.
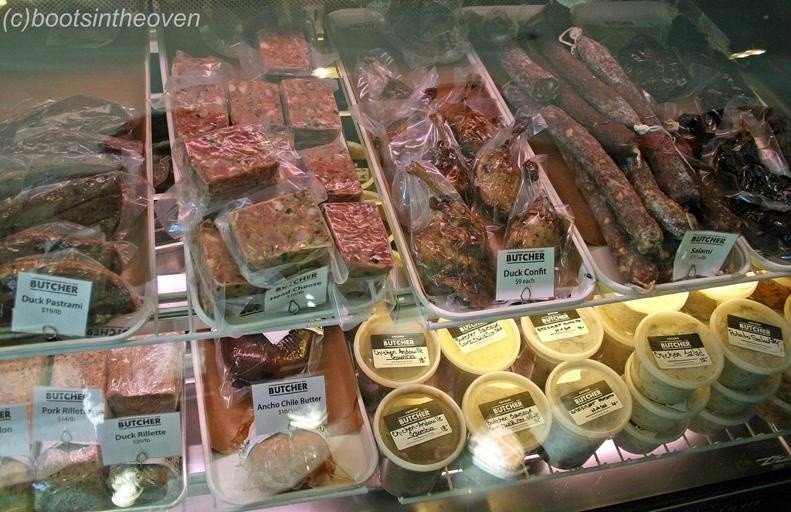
494;34;744;286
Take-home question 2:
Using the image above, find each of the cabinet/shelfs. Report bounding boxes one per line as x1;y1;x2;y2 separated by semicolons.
2;1;791;510
